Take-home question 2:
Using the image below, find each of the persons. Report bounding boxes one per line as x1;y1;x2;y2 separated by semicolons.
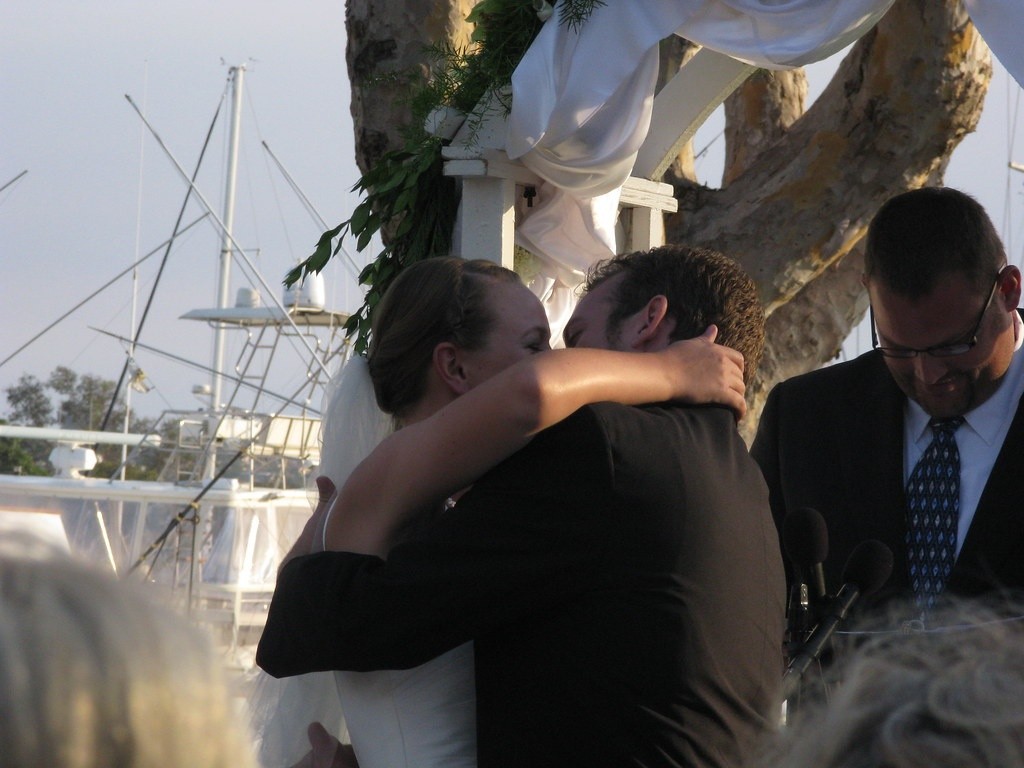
739;185;1024;684
761;598;1024;768
254;246;788;768
245;255;747;768
0;533;243;768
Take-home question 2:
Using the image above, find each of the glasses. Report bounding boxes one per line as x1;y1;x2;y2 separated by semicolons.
870;262;1007;359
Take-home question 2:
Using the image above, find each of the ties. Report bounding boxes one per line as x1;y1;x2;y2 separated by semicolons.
904;414;965;626
